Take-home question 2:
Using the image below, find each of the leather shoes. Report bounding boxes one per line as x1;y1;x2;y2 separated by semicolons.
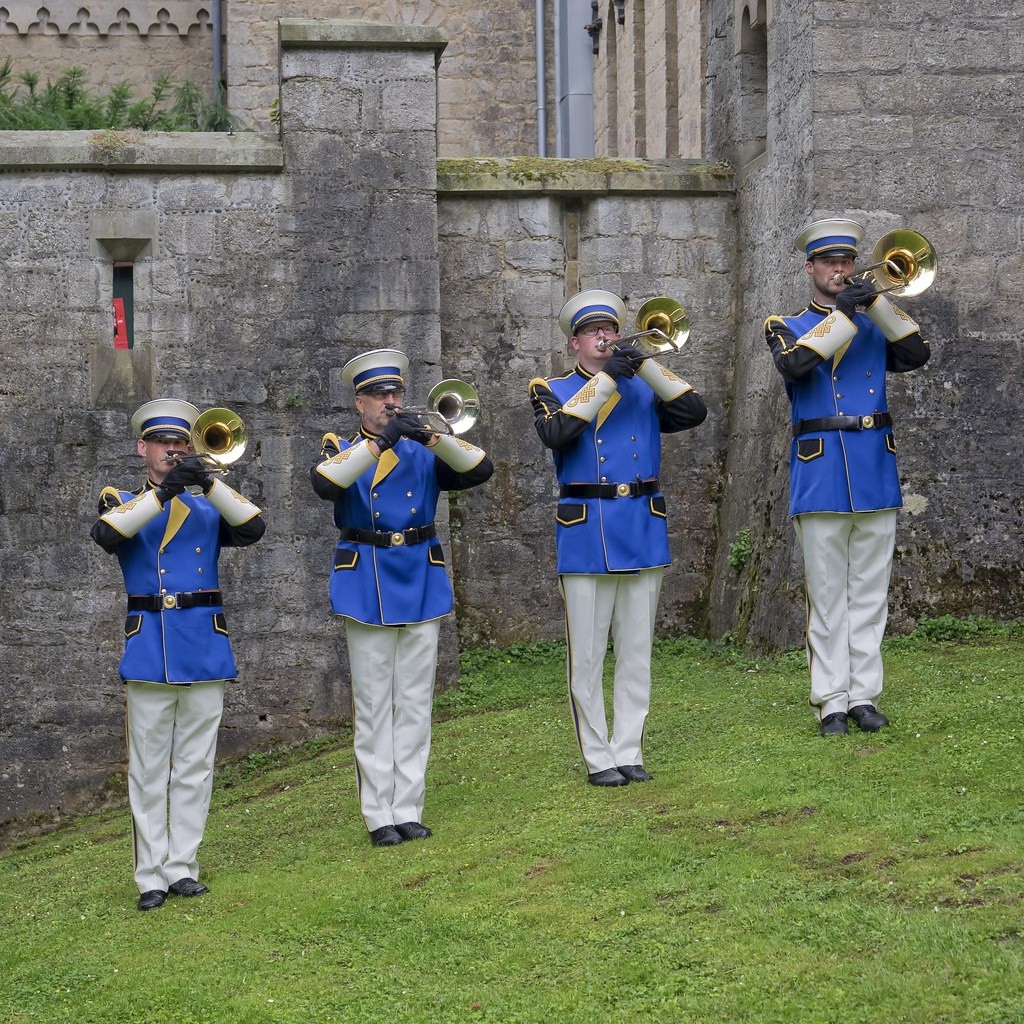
368;824;403;846
168;878;209;896
587;768;628;786
617;764;653;782
136;890;167;910
821;712;849;737
396;821;433;842
848;705;888;731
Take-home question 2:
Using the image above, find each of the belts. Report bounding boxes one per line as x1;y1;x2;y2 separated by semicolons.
792;411;895;436
129;591;224;612
559;479;662;499
339;524;436;548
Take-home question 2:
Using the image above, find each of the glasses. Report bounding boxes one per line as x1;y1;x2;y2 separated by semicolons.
577;325;618;336
359;388;406;400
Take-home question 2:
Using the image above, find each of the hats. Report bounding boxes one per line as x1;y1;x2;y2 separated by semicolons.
340;349;410;393
795;218;866;261
559;289;627;336
131;397;202;444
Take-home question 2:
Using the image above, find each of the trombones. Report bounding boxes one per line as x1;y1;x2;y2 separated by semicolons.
832;228;940;299
385;378;480;436
597;296;690;362
167;406;251;477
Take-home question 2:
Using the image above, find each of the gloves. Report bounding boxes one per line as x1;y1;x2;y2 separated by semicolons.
159;450;213;497
601;343;644;380
836;279;880;320
372;405;433;453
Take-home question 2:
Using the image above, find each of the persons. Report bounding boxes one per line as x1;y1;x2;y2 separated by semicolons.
91;398;267;910
310;349;495;847
764;217;931;736
529;290;707;787
